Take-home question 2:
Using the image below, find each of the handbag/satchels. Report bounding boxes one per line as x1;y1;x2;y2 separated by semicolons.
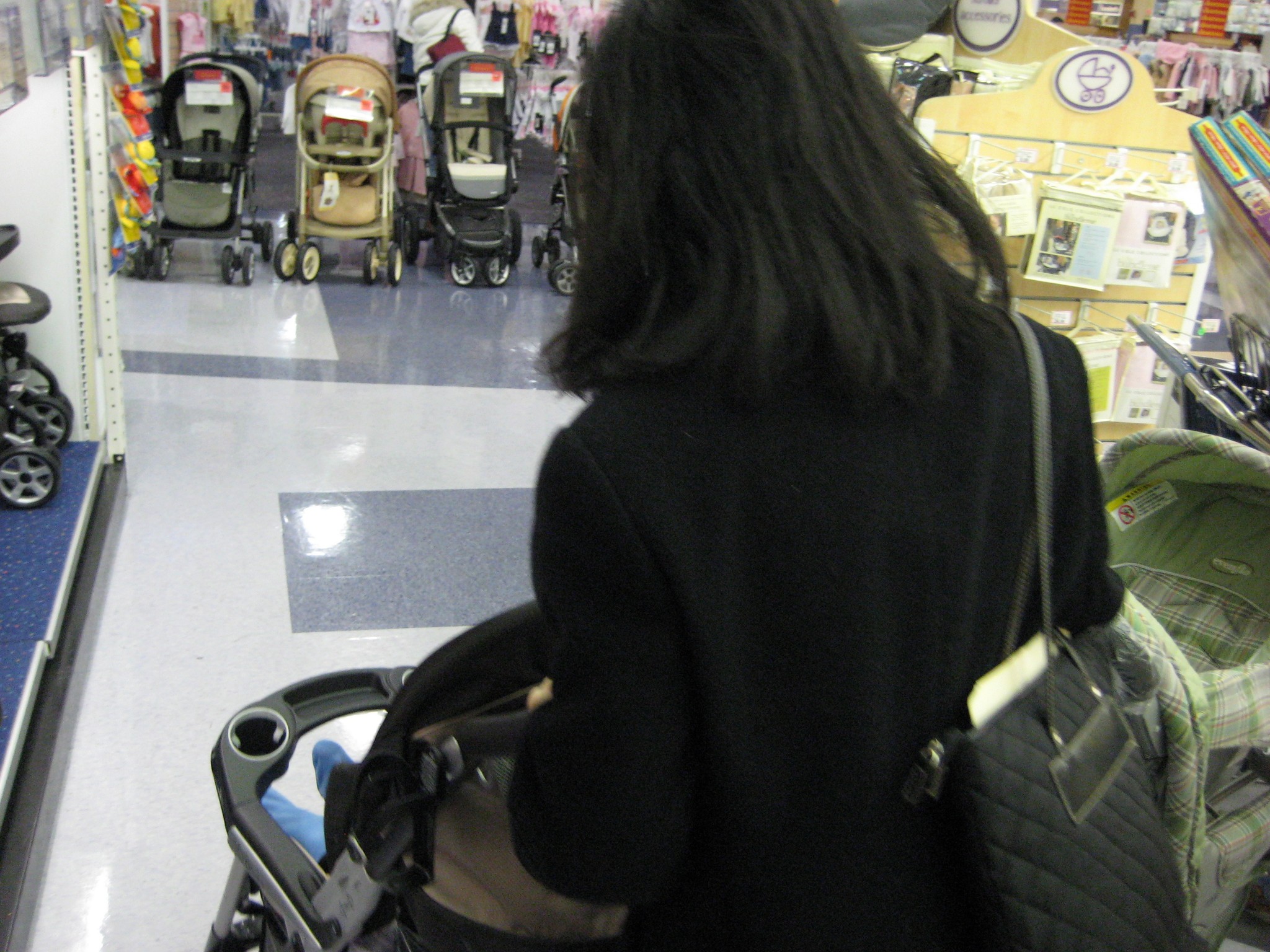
901;315;1211;952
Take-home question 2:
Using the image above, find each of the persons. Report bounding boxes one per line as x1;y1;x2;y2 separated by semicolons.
507;0;1125;952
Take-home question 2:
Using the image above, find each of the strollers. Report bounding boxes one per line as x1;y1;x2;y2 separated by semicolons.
535;70;591;295
268;54;410;288
1065;428;1268;921
396;50;528;290
200;601;641;950
135;46;278;288
1127;308;1270;448
0;224;75;509
